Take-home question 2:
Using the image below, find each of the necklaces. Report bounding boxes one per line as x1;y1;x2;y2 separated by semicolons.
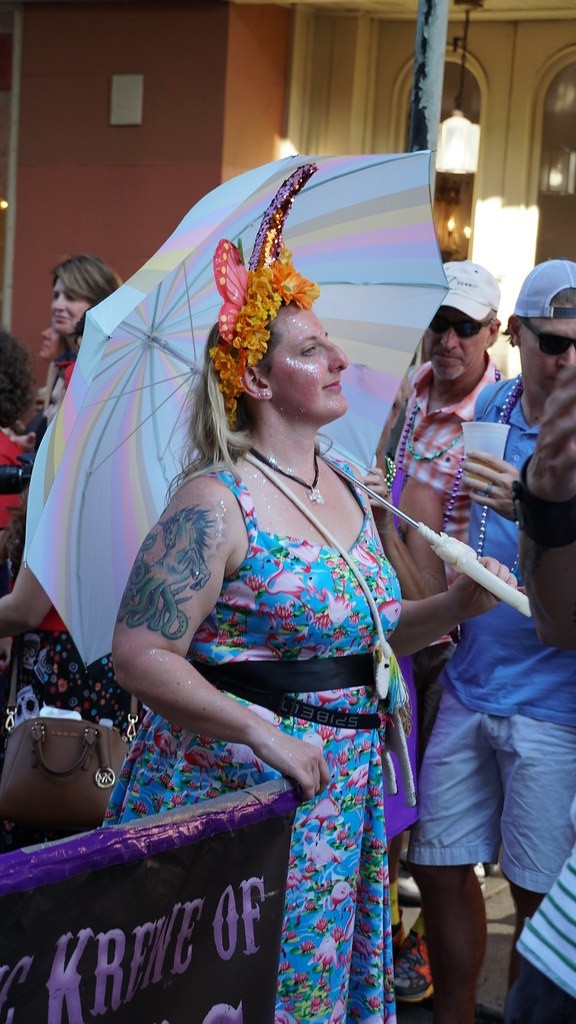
385;366;502;530
477;374;523;573
248;448;322;505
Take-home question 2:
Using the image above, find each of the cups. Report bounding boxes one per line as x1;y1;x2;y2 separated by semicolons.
462;422;510;485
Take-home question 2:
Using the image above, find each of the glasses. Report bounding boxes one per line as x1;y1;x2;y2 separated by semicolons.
429;314;497;338
515;315;576;356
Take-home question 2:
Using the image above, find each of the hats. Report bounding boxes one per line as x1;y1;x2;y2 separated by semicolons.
513;257;576;324
441;260;501;320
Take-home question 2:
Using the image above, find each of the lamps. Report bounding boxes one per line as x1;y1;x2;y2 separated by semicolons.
433;0;486;175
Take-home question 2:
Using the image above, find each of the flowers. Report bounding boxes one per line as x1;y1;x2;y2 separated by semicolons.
209;260;320;430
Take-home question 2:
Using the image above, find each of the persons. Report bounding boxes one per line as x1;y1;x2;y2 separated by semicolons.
364;261;503;999
505;364;576;1024
103;242;520;1024
0;253;138;853
398;261;576;1024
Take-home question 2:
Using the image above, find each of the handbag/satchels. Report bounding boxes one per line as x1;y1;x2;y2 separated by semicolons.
1;714;130;829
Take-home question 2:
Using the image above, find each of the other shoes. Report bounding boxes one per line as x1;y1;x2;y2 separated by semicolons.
394;846;486;900
391;926;435;1003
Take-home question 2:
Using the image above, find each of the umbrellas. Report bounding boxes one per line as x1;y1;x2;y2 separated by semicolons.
22;149;531;676
